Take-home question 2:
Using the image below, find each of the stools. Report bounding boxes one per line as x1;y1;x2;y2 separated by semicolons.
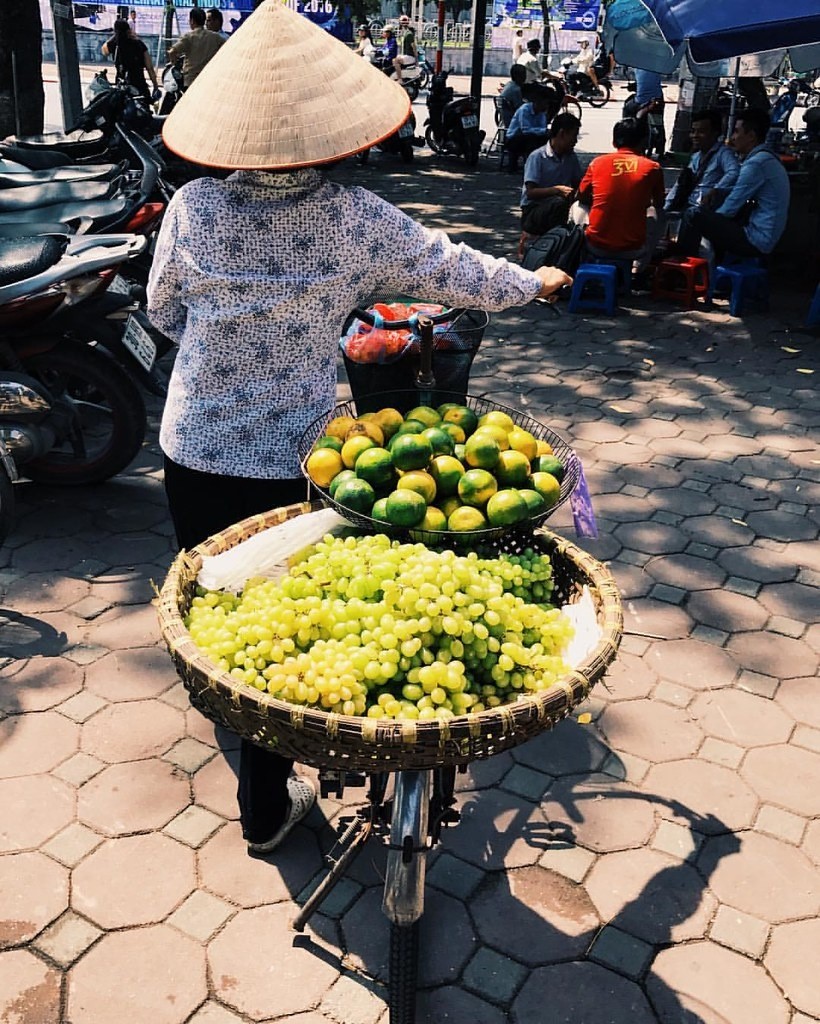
568;258;633;318
804;282;820;327
499;145;528;168
517;231;539;260
645;248;770;317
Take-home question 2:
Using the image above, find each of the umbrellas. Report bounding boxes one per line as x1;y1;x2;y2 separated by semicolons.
606;1;819;152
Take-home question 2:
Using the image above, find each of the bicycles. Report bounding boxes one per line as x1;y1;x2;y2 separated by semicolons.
283;287;599;1007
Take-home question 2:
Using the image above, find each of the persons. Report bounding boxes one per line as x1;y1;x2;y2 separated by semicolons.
128;9;136;36
619;65;671;155
519;108;790;304
167;7;230;89
499;26;555;163
144;1;572;855
100;19;162;117
571;34;608;96
353;25;422;84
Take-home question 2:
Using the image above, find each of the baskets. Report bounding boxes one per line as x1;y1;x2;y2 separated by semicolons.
157;501;627;769
298;398;584;542
337;296;490;416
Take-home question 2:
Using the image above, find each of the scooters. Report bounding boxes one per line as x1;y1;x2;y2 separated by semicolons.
0;69;178;499
356;60;428;166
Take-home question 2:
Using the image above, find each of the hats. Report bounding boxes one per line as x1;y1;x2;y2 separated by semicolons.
161;0;412;171
576;36;590;43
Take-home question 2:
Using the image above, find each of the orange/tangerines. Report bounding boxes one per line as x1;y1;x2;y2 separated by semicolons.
306;403;564;542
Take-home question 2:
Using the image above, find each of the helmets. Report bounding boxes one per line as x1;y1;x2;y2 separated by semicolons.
399;15;410;21
527;38;540;48
357;24;369;31
381;25;395;34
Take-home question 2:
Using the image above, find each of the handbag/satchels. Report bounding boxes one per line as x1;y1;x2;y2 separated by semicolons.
520;223;585;272
339;301;472;388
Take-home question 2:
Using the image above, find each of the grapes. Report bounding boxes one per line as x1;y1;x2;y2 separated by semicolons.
186;535;572;721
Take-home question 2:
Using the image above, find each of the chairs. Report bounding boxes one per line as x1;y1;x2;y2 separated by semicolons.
486;96;515;167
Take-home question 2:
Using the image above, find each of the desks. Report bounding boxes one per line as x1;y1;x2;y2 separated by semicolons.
668;151;797;170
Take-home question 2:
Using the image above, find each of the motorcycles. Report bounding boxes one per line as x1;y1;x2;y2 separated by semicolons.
494;68;589;127
555;56;613;110
423;66;488;163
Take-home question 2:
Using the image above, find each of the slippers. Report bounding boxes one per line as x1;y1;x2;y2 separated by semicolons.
247;775;315;854
